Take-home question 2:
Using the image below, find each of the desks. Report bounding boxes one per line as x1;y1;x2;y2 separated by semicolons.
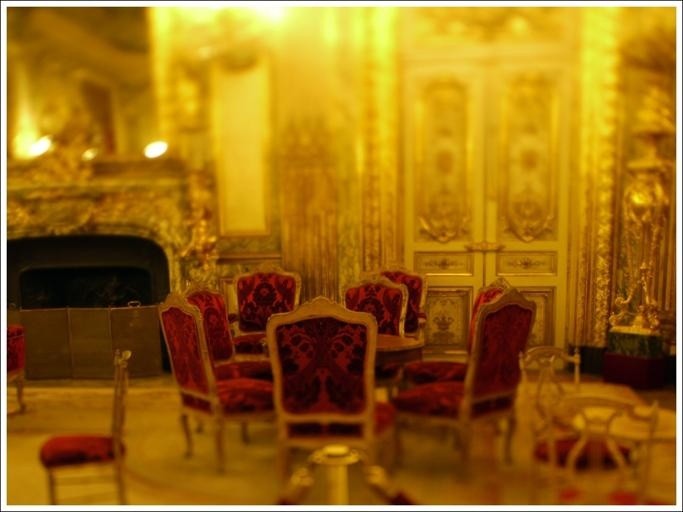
580;404;674;503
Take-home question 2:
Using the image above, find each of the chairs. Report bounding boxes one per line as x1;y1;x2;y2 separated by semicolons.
7;324;28;412
159;267;537;502
546;396;660;503
517;345;582;445
39;350;132;506
